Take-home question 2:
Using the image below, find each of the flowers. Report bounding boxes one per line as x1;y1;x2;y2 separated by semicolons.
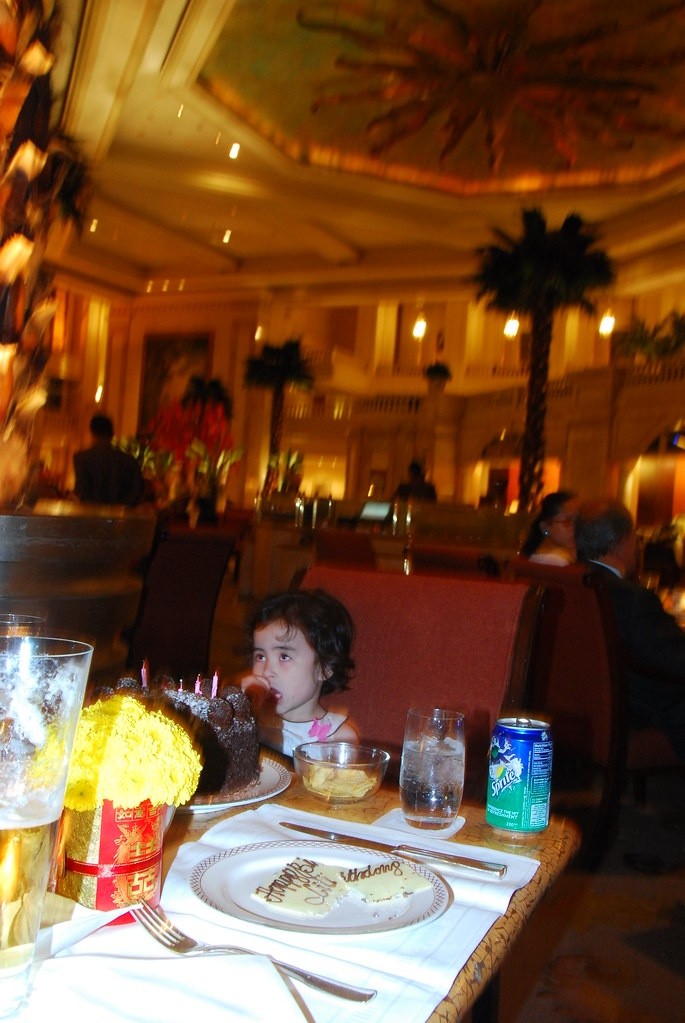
24;692;198;814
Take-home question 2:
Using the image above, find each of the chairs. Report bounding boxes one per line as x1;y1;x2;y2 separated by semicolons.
400;544;499;581
314;528;374;567
507;557;682;854
121;534;235;683
254;560;542;782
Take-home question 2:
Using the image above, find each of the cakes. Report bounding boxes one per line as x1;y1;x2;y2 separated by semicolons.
91;677;260;797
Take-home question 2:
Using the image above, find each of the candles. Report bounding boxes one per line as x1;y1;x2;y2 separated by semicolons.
141;661;148;689
210;671;219;698
193;673;201;693
178;679;184;692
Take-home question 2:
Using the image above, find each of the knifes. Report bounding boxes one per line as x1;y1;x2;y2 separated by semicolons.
278;821;507;881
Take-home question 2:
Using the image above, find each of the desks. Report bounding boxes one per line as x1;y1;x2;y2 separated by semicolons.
0;769;579;1023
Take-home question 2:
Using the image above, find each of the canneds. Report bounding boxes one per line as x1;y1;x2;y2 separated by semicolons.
486;717;553;834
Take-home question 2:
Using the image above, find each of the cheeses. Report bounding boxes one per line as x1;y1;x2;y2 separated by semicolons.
252;858;434;915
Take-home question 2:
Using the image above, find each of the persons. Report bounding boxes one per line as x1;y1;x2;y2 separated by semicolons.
574;494;685;765
394;462;436;503
644;514;685;591
520;492;579;565
73;412;145;504
239;589;361;767
22;461;61;508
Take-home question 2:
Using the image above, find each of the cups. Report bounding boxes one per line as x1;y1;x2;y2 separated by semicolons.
0;613;46;638
398;708;466;830
0;636;94;1023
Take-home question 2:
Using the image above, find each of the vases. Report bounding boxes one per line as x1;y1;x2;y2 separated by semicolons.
53;804;165;923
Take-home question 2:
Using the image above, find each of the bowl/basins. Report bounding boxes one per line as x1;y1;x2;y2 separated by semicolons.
293;740;390;805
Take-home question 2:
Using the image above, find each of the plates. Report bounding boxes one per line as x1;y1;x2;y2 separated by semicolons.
175;757;292;815
187;840;449;937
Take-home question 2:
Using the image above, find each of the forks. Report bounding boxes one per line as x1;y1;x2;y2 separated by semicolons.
128;897;377;1004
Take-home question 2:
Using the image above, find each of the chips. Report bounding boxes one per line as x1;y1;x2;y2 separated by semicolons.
302;765;378;798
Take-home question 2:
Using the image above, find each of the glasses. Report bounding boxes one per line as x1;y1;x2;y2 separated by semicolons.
551;516;575;529
